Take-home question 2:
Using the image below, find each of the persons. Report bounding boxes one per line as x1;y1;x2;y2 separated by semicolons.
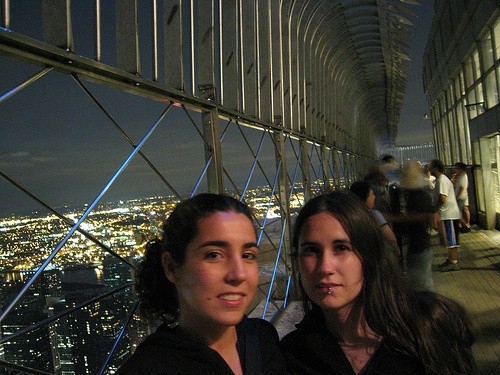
427;158;463;273
363;153;474;261
114;191;289;375
281;189;480;374
349;180;401;256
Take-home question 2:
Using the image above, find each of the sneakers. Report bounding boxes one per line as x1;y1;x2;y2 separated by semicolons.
439;259;461;272
